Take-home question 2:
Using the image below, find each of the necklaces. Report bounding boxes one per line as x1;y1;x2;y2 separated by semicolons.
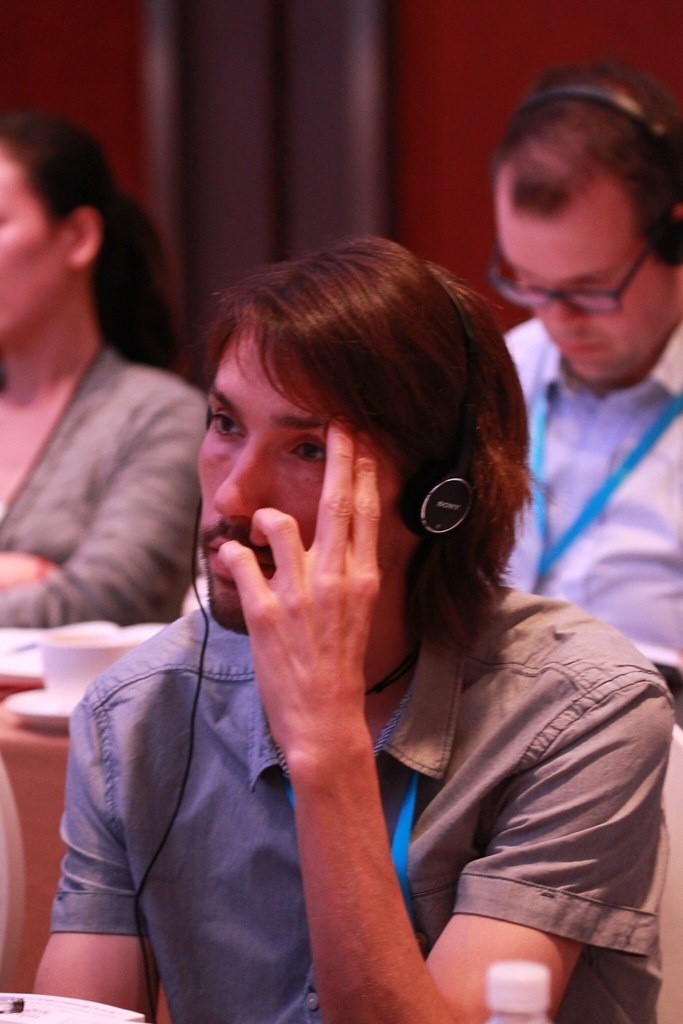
366;644;418;697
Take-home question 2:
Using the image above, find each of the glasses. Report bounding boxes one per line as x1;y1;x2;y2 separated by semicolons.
488;220;667;311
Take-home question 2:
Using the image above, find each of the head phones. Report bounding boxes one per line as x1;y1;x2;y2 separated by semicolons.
399;271;481;539
509;87;683;265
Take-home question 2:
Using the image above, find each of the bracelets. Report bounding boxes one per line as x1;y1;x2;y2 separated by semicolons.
35;555;48;584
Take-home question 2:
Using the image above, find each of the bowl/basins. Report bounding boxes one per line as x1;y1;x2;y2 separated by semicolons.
38;623;171;704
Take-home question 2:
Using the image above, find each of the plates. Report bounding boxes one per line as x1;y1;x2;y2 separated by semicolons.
6;689;68;729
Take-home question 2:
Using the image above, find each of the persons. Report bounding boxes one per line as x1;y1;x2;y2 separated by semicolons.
0;110;206;629
29;238;675;1024
485;61;683;729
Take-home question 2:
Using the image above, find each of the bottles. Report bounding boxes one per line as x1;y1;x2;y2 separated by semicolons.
480;960;557;1024
